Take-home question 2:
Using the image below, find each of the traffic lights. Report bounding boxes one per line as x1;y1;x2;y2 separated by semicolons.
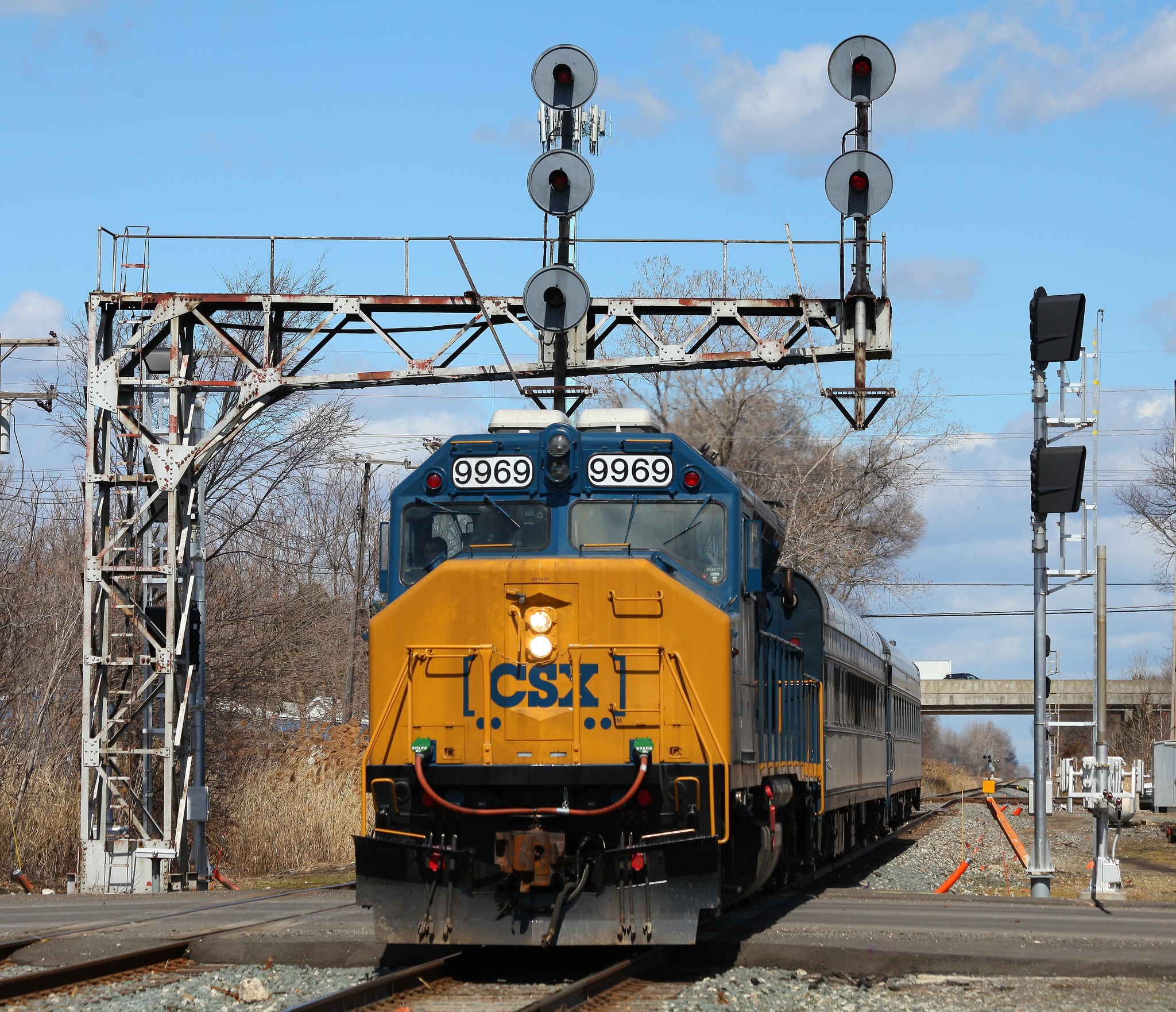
828;34;896;104
527;149;592;218
524;265;590;335
531;43;598;111
1029;437;1089;515
1029;287;1087;364
825;149;895;217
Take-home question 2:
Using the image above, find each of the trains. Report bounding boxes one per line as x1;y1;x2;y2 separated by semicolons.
349;406;923;949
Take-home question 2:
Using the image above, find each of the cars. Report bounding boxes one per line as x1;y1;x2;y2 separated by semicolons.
1139;783;1166;811
942;673;981;679
